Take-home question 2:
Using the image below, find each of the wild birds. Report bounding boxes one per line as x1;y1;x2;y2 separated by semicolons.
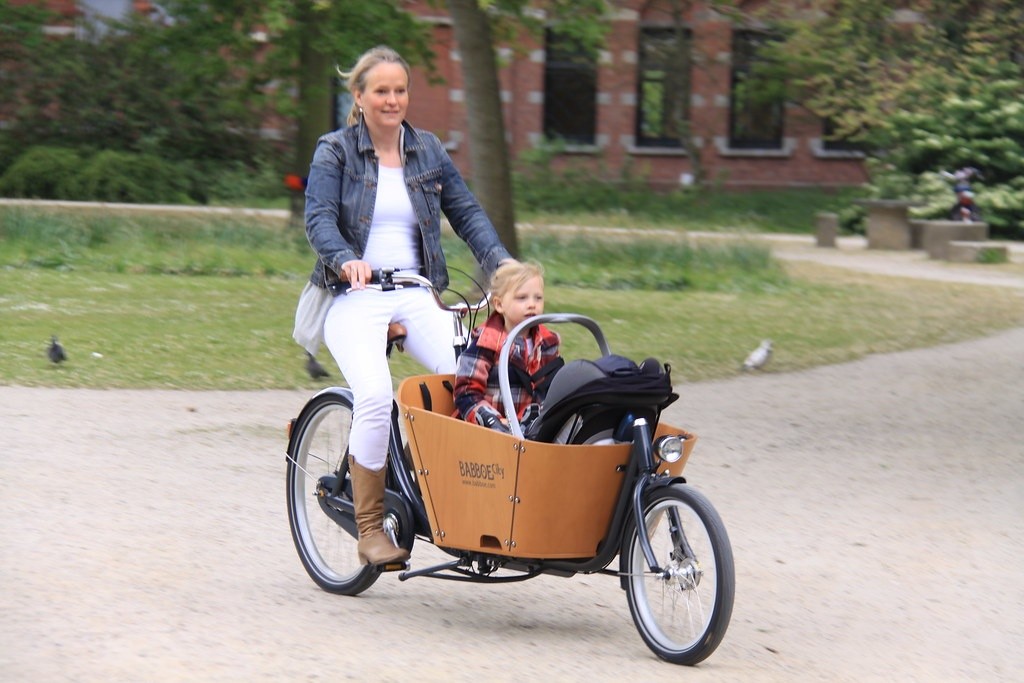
46;336;67;363
744;340;775;371
308;355;331;380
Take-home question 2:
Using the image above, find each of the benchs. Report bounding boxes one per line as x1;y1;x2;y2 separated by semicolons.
909;219;990;251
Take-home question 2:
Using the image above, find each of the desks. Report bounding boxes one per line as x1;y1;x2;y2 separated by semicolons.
853;199;928;250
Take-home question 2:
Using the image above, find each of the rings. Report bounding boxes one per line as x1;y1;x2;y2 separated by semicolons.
351;268;357;272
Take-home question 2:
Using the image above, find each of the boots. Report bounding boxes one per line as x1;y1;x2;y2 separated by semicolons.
348;453;410;566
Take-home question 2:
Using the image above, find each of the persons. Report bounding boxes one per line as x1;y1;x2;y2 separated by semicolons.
451;261;563;441
291;44;518;565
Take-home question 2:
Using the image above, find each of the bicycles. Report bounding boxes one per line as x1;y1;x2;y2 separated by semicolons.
943;171;980;223
284;265;737;668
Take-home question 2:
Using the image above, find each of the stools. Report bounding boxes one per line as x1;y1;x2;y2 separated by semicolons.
815;212;838;247
